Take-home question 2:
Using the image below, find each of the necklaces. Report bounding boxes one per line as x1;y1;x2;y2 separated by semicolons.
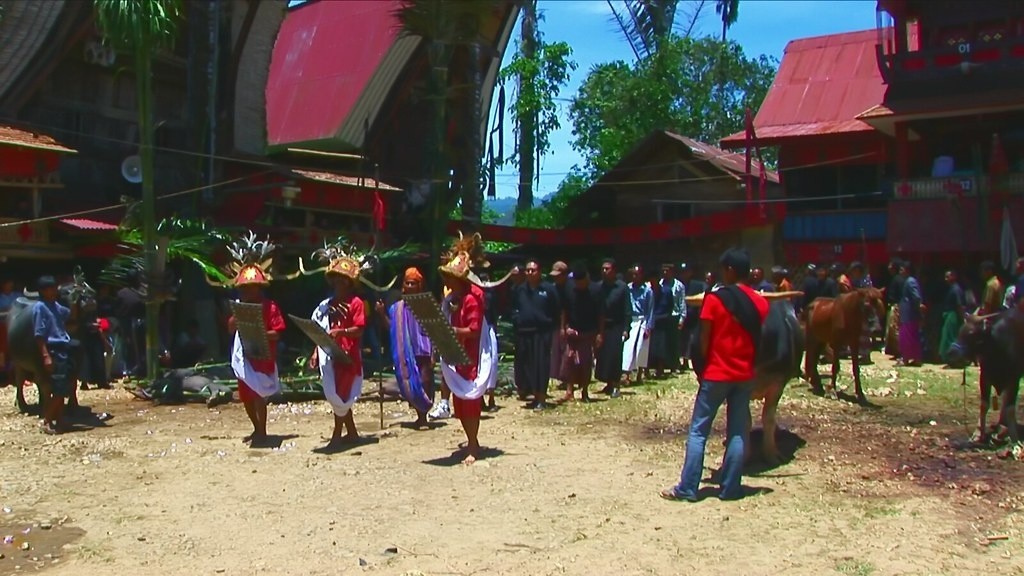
448;287;467;313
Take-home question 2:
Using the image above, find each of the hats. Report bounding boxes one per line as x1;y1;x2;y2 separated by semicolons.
298;241;397;291
438;231;512;287
549;262;568;278
205;231;301;290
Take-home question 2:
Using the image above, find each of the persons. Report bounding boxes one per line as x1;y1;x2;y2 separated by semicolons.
228;243;1023;503
0;274;209;434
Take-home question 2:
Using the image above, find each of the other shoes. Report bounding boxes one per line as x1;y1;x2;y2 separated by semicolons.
660;488;691;501
488;400;497;414
528;362;688;412
42;420;63;435
429;407;451;419
894;360;922;367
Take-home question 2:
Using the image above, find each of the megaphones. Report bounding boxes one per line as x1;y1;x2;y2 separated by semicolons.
121;155;142;183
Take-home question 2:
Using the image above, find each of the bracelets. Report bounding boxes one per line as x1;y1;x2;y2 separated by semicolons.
452;326;457;335
344;328;350;336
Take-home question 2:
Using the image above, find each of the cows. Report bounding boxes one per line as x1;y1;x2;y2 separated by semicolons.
683;290;805;463
948;308;1024;448
6;266;100;420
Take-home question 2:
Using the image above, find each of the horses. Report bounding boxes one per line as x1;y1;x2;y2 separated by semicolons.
805;287;887;401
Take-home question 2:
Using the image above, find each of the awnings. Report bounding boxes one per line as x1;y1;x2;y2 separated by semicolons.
50;210;167;236
0;124;78;154
292;169;405;192
